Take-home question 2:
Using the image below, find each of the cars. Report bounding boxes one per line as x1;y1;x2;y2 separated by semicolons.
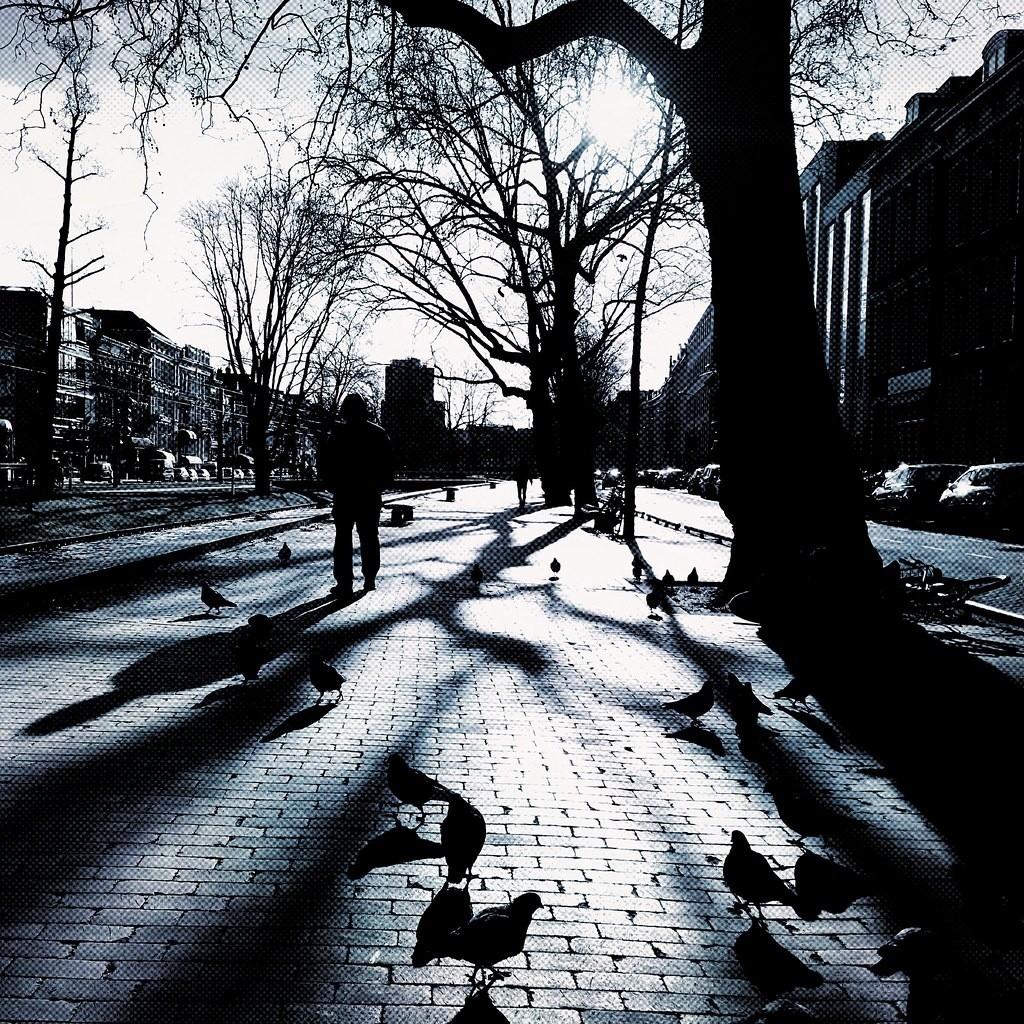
234;468;245;480
197;469;211;481
869;462;971;528
173;467;190;481
601;470;625;490
593;469;602;479
938;461;1024;533
80;462;113;485
187;469;199;483
636;464;722;500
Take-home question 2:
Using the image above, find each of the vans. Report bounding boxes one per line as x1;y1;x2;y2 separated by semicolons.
144;458;177;483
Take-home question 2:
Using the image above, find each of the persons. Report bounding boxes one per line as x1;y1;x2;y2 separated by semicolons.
514;459;532;505
323;393;395;597
644;469;650;488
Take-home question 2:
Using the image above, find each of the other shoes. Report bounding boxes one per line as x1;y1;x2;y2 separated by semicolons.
330;581;354;600
364;577;376;590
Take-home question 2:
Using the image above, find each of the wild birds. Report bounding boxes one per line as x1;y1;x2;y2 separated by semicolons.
442;792;486;884
662;570;675;590
632;553;647;583
646;588;661;610
687;567;699;586
763;779;871;845
773;676;816;714
248;614;278;635
726;671;774;722
234;638;275;685
721;829;799;914
551;558;560;572
661;681;716;726
308;652;347;704
435;893;546;984
198;580;238;614
387;754;451;821
276;542;291;561
471;564;484;585
